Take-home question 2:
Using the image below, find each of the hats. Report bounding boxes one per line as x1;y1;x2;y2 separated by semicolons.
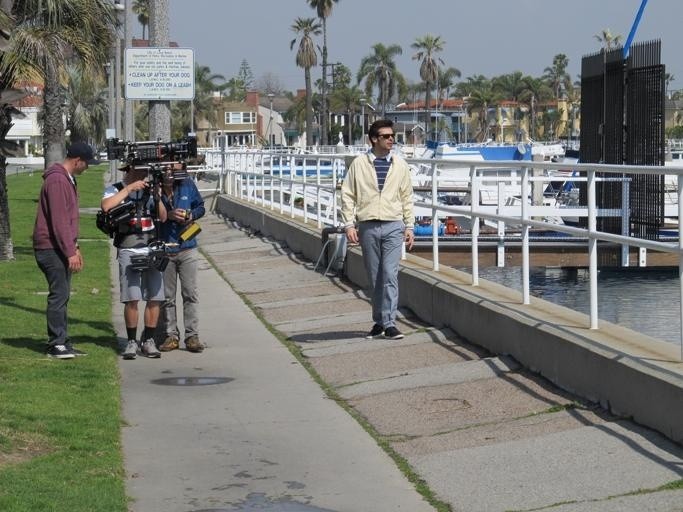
67;143;100;165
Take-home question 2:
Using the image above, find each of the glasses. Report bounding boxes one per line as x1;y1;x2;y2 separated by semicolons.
378;134;395;138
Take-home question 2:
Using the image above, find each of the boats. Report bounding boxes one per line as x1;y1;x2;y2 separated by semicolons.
270;138;566;236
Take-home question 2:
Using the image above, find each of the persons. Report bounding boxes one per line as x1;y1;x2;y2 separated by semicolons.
340;119;417;339
100;160;169;360
151;173;205;353
31;142;102;360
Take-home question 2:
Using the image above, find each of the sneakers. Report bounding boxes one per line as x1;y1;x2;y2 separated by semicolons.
186;336;203;350
159;337;178;351
141;338;161;358
65;342;88;356
123;339;138;359
385;327;404;340
366;324;384;338
47;344;74;358
